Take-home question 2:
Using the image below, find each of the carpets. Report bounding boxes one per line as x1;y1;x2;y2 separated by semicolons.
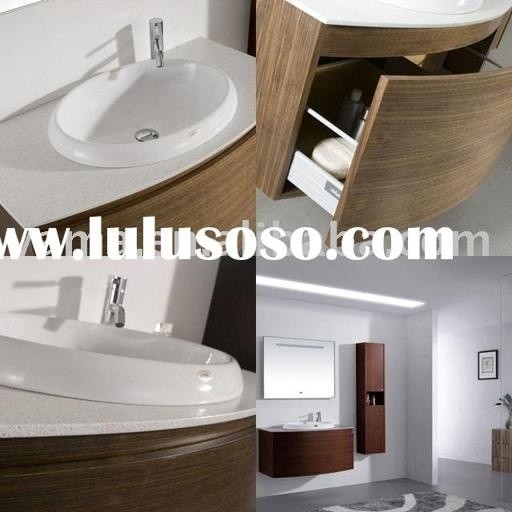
301;490;512;512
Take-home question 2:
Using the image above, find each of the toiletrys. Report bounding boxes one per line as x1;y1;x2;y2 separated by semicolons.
352;105;369;140
337;88;363;132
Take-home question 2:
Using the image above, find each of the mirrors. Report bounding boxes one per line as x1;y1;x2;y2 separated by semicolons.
263;336;335;400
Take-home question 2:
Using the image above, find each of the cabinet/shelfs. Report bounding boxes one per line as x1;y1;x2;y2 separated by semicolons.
491;429;512;472
355;343;385;454
256;0;512;249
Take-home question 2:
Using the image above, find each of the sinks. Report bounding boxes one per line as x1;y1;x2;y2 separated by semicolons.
0;310;243;403
50;59;237;166
283;422;335;429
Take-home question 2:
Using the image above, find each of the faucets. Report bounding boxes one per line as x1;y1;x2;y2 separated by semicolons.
148;17;165;68
103;275;127;328
316;412;321;422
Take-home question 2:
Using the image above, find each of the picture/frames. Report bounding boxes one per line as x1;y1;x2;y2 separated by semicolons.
476;349;499;380
